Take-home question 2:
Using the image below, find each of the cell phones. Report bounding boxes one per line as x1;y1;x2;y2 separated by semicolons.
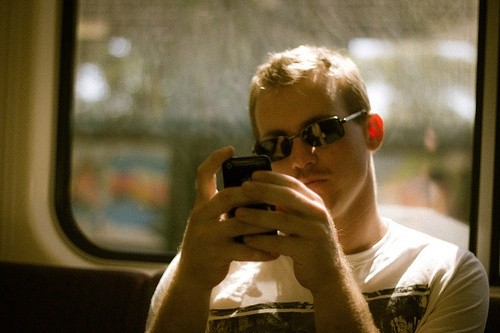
222;156;278;244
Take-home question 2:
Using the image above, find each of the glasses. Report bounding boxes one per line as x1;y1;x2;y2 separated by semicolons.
251;110;367;162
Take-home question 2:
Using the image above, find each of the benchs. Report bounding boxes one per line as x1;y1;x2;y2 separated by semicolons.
0;260;500;333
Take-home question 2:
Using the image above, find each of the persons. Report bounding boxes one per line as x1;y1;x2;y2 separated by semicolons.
145;44;489;333
